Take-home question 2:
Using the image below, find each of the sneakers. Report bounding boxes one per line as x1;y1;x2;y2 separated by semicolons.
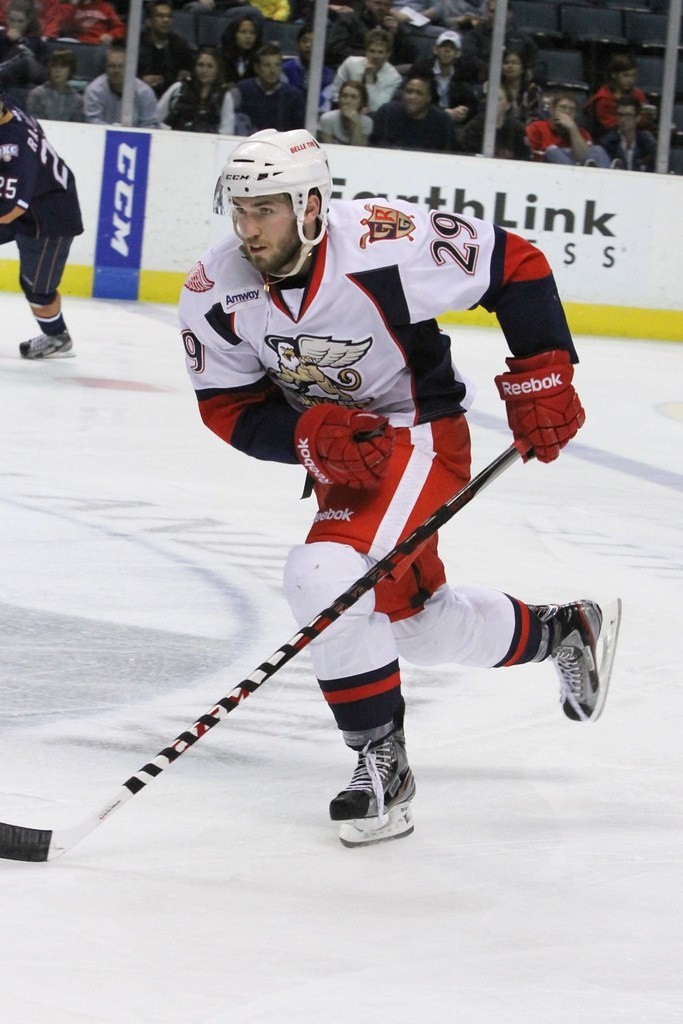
532;595;620;724
19;329;78;360
329;698;416;846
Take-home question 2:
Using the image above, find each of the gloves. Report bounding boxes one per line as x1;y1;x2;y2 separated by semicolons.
494;349;585;463
295;404;395;492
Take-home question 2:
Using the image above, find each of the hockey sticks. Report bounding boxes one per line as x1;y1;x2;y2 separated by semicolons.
0;437;539;864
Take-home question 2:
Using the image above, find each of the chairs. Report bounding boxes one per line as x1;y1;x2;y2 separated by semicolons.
0;0;683;177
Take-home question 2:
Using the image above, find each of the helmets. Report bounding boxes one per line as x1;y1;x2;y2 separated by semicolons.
213;124;332;244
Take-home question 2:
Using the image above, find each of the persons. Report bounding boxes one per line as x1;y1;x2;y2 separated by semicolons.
222;18;263;77
316;82;374;146
326;24;404;113
372;70;458;154
177;125;623;852
423;1;512;28
135;5;189;78
2;92;84;360
155;47;235;138
579;52;657;129
184;1;253;17
230;44;308;134
0;0;159;129
484;50;545;120
327;1;414;71
394;29;478;123
457;82;531;163
524;97;622;170
602;97;659;174
284;23;337;92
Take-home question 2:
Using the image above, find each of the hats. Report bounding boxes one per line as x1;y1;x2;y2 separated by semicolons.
437;30;464;49
